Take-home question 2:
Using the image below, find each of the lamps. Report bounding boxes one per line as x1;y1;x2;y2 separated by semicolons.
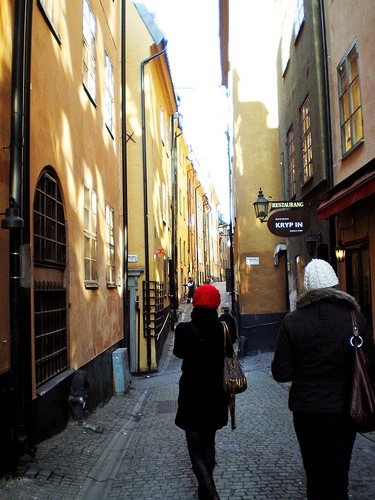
251;187;271;222
333;219;357;262
305;229;322;258
0;208;25;229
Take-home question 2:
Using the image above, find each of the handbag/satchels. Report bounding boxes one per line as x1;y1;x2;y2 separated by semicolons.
221;321;247;394
350;310;375;434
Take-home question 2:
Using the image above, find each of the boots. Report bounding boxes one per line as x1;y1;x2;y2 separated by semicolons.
192;460;221;500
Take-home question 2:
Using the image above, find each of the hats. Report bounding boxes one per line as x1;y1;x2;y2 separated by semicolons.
191;284;221;308
303;258;339;291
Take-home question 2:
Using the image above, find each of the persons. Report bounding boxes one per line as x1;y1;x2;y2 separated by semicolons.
271;258;375;500
172;275;238;500
